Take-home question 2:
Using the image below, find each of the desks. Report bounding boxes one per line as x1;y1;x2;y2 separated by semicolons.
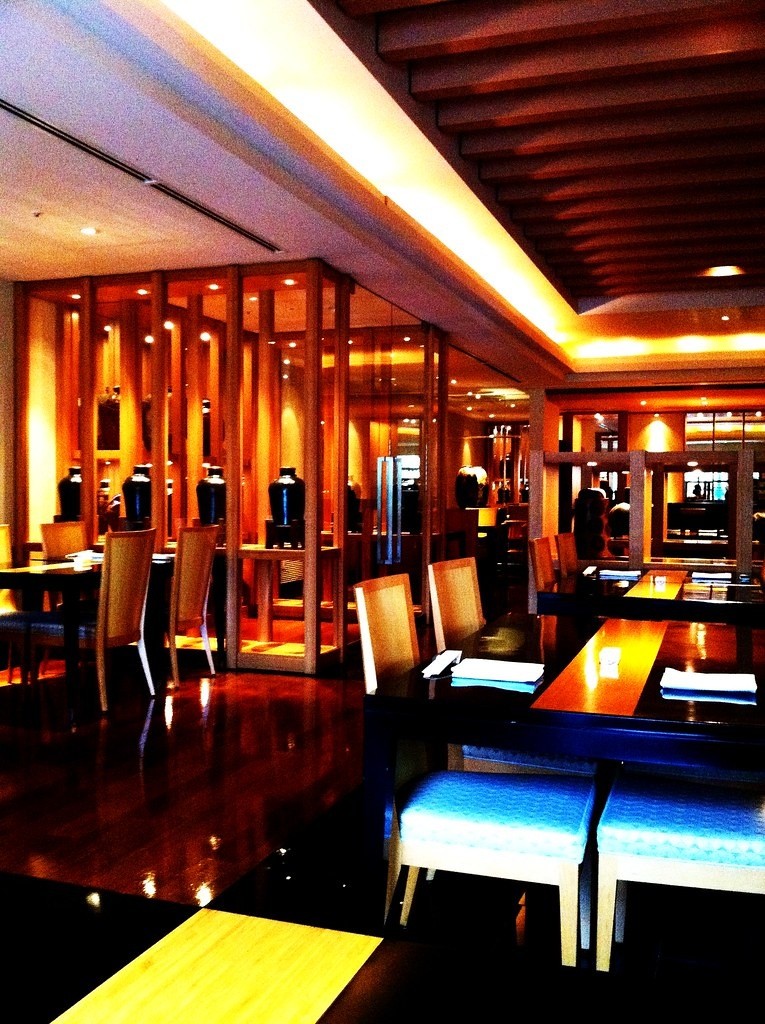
319;530;442;562
536;566;765;613
0;870;385;1024
0;548;227;696
375;610;765;784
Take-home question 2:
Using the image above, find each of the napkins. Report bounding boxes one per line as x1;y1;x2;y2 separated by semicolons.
659;688;757;706
599;570;642;577
692;572;732;579
599;575;638;581
152;554;171;560
450;678;543;694
451;657;544;683
660;666;758;693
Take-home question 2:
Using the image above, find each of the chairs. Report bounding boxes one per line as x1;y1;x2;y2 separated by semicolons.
111;524;221;688
0;524;30;684
595;776;765;973
554;532;579;577
528;536;556;592
40;519;90;675
351;573;597;967
426;556;603;778
29;527;158;711
